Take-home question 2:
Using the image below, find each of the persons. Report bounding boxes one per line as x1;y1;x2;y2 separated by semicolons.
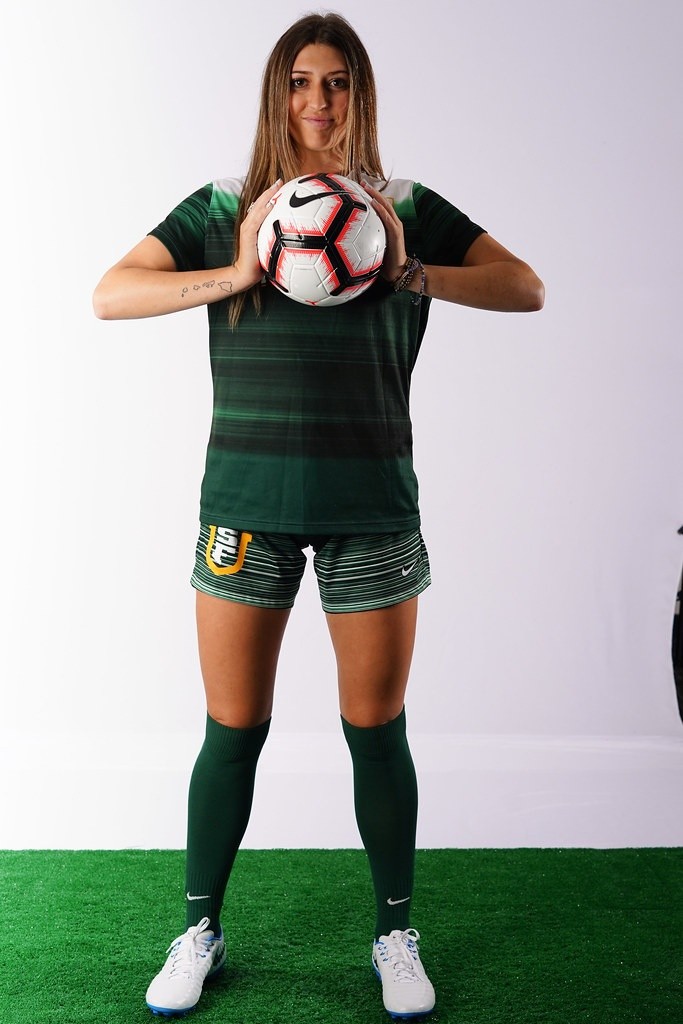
92;12;545;1014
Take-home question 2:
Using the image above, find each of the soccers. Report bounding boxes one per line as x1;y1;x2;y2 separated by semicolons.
256;171;388;308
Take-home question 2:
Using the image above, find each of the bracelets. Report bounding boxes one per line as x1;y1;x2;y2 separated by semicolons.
392;257;426;306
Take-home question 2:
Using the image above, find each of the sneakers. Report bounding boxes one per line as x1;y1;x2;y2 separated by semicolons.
371;932;437;1015
145;926;228;1015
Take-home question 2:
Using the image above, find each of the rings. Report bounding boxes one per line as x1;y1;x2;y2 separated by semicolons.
246;201;255;213
